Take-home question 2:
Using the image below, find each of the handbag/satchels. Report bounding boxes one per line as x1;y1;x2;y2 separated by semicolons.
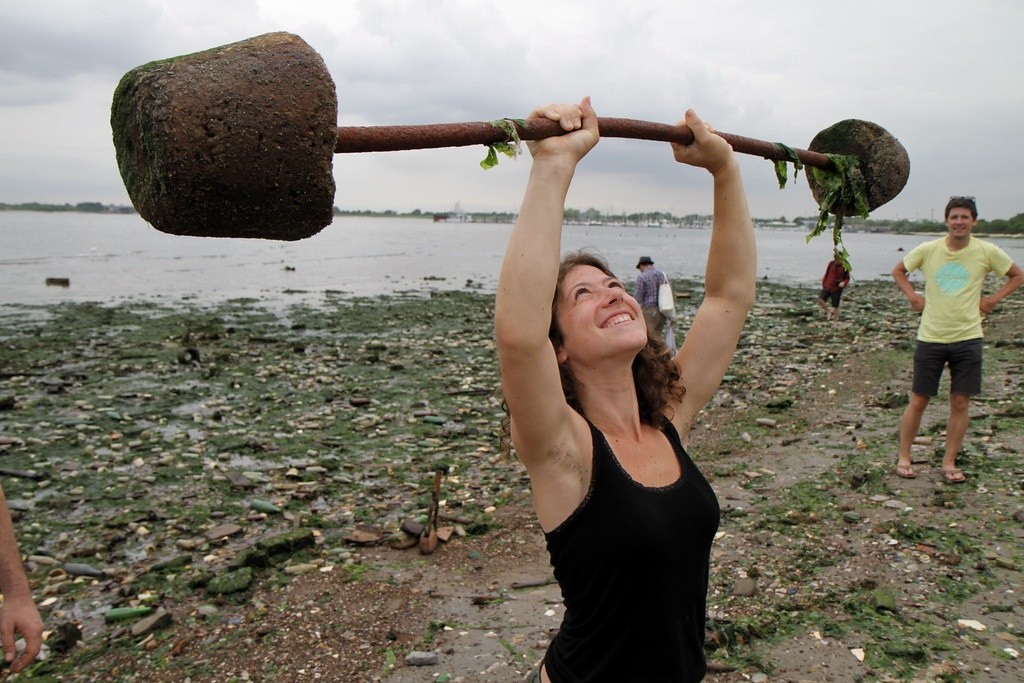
664;319;676;357
658;272;676;322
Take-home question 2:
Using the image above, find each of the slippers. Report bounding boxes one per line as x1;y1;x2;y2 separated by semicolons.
941;469;965;483
896;465;917;478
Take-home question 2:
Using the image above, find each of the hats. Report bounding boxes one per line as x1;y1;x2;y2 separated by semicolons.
636;257;654;269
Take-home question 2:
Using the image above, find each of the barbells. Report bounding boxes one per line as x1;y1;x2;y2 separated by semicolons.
109;31;912;241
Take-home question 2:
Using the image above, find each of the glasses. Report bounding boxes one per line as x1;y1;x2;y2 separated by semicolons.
950;195;975;207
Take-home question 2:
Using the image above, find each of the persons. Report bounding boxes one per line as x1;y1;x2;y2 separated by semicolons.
494;96;758;683
634;256;677;337
0;482;44;673
892;196;1024;484
819;251;850;322
